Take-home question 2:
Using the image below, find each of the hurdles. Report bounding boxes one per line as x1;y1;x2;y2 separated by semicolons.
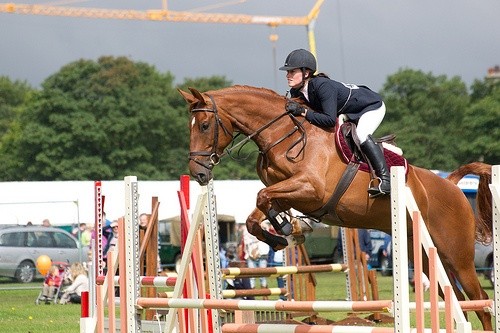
81;175;496;333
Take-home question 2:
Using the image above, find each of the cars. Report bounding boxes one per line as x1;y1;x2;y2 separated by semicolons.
104;219;183;276
0;226;89;284
299;217;343;265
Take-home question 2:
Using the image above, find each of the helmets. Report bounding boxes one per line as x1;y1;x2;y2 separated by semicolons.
279;49;316;71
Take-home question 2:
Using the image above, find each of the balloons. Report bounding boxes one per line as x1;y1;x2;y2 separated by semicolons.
37;254;51;276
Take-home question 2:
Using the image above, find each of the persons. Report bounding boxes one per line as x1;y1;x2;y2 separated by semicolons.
278;49;392;197
22;212;372;305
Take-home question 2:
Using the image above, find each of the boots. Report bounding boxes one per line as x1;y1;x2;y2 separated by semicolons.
360;134;391;194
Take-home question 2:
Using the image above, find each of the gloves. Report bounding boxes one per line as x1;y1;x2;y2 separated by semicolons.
286;101;305;117
